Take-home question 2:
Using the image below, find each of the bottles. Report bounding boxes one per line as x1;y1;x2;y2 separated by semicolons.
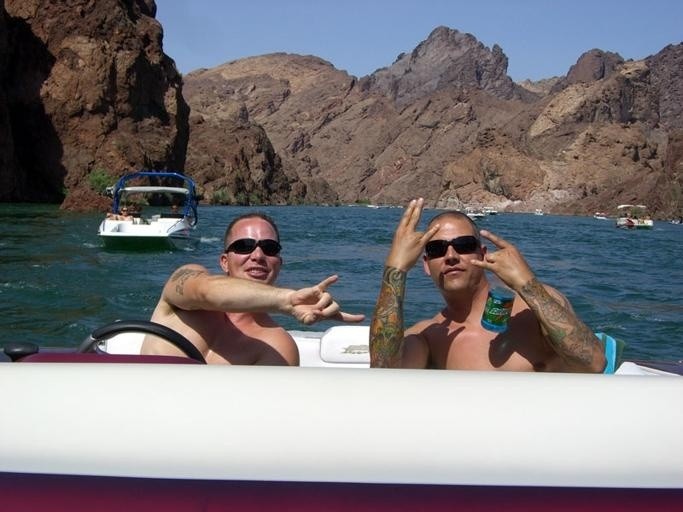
480;266;516;333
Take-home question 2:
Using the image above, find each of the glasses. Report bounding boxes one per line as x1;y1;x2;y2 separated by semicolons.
223;238;280;258
424;234;481;259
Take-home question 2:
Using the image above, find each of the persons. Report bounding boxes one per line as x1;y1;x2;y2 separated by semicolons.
620;212;650;220
169;205;179;213
366;195;609;374
139;212;366;366
107;201;143;221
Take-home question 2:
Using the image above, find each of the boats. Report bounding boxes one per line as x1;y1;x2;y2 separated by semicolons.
593;213;607;220
0;314;683;510
481;207;498;214
616;204;654;229
534;209;543;216
464;206;485;217
95;171;201;252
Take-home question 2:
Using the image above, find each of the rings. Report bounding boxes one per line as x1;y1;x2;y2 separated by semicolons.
487;254;496;262
